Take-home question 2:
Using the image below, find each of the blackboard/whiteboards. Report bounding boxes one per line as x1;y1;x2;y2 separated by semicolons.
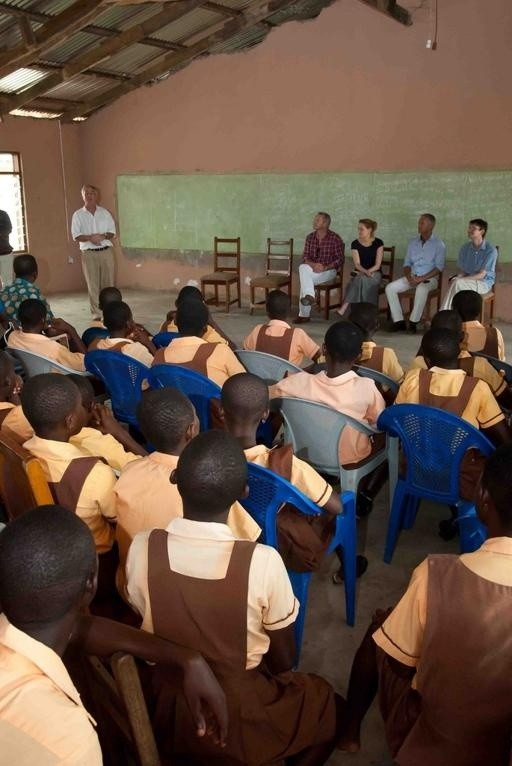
117;172;512;263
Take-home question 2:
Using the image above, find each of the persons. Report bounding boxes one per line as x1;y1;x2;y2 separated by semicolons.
437;217;499;314
2;503;227;765
326;452;512;765
69;182;118;323
390;289;511;538
383;211;448;333
0;282;407;622
333;217;385;320
0;253;56;336
121;427;348;764
291;210;346;325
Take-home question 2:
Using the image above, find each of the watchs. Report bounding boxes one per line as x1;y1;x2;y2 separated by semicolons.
104;232;108;238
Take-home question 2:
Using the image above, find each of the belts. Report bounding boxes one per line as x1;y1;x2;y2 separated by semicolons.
88;246;109;251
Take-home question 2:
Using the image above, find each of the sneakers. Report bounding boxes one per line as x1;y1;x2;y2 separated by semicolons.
389;321;407;332
407;321;417;334
355;491;373;520
332;556;368;585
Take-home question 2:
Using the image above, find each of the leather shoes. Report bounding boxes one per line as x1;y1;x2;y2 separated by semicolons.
301;295;318;305
292;317;310;323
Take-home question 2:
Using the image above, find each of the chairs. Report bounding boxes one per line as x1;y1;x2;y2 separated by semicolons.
478;245;501;326
200;237;240;313
376;403;496;567
376;246;396;319
249;237;293;313
399;271;443;328
1;329;512;526
268;397;399;529
75;645;163;765
310;244;345;321
238;459;356;670
